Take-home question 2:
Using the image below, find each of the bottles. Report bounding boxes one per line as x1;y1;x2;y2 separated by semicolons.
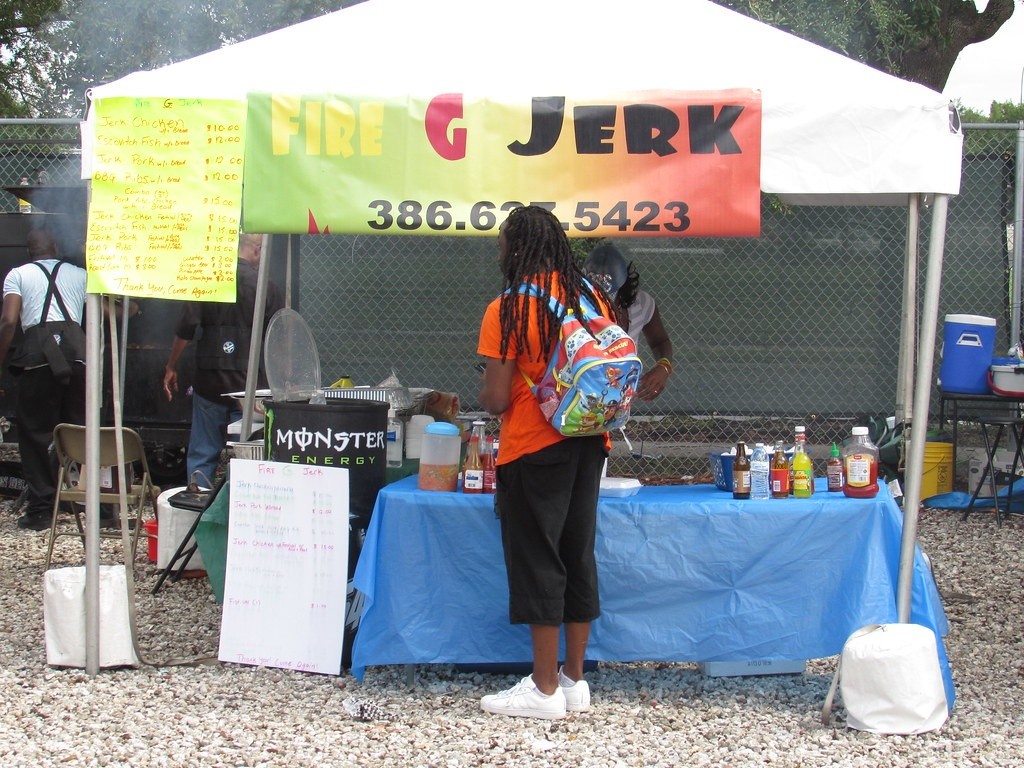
827;429;880;499
731;426;815;499
384;410;403;469
418;422;500;494
329;374;354;389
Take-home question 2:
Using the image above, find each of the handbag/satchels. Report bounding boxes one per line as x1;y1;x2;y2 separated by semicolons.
821;623;948;736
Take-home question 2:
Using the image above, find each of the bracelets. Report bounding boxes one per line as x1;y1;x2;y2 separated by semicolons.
657;358;672;376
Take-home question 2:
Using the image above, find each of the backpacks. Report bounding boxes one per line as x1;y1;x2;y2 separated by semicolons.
497;277;642;437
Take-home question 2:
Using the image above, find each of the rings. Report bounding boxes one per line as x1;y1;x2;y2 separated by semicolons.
654;390;657;393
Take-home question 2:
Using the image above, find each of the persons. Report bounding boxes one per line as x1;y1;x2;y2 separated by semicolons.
582;242;672;401
478;207;616;717
163;232;281;486
0;229;138;529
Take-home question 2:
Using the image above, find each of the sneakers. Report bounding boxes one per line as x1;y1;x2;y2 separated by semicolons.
480;674;567;720
563;680;590;712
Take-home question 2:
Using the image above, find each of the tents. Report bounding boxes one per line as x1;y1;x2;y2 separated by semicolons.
79;0;963;674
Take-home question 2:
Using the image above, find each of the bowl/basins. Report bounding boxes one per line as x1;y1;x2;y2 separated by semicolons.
229;395;272;422
405;414;435;459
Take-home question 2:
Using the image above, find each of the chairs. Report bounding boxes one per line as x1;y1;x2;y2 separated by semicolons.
45;423;156;573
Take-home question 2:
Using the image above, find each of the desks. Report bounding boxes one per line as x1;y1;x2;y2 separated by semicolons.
351;475;955;710
940;391;1024;491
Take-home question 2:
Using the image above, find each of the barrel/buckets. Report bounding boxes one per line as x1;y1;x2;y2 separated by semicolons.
142;518;157;563
935;314;1024;399
919;442;954;501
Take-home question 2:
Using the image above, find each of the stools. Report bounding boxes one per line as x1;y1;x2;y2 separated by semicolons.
961;415;1024;529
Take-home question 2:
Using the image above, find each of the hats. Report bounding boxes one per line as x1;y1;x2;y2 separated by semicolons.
583;246;628;294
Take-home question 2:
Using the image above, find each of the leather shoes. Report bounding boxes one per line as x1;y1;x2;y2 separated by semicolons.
17;511;54;530
